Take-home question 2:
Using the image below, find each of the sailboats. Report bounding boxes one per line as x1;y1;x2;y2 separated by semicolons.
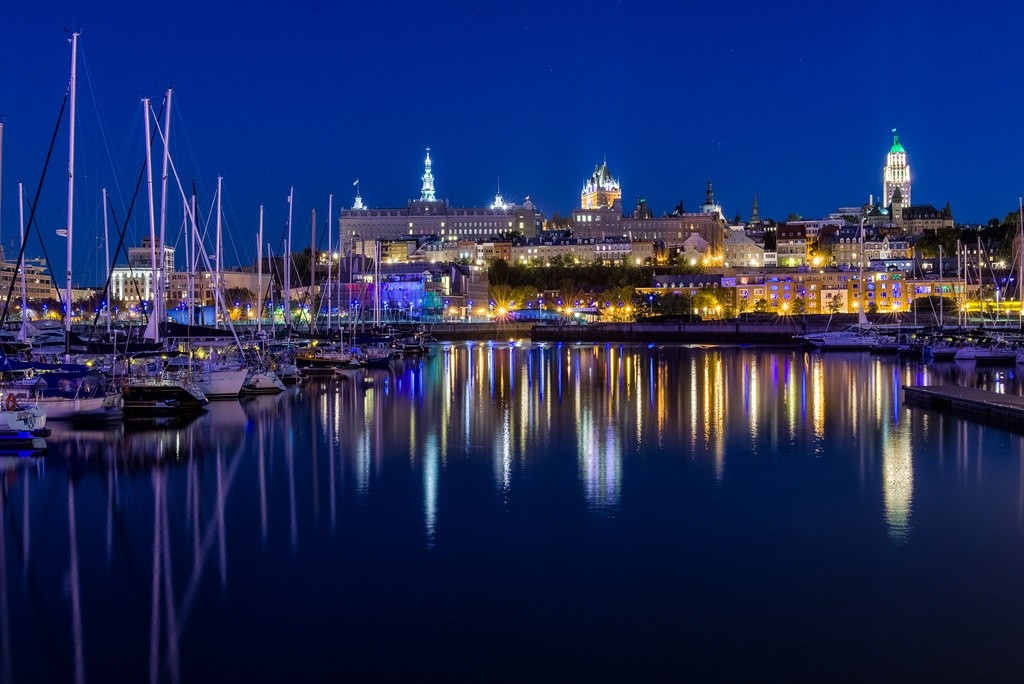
1;27;441;448
789;198;1024;360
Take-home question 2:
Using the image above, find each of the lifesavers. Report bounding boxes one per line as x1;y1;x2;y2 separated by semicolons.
5;395;16;411
115;378;128;392
